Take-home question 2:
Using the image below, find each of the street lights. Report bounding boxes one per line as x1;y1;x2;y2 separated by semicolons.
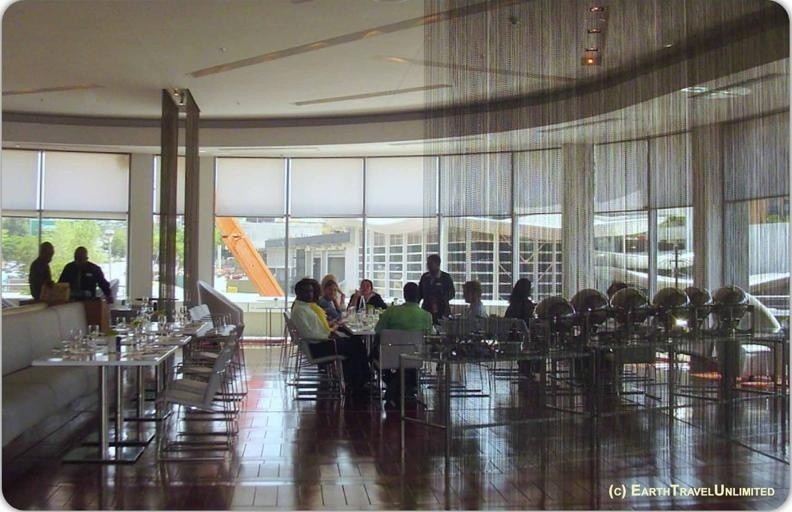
104;229;115;280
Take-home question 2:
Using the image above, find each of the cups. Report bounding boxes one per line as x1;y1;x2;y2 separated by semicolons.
59;296;191;357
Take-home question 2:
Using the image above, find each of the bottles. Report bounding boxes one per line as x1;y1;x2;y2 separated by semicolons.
340;295;403;332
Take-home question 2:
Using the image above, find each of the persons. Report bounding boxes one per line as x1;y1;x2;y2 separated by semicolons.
503;277;542;379
290;273;436;411
445;279;488;339
58;246;116;305
416;253;457;322
28;241;57;303
